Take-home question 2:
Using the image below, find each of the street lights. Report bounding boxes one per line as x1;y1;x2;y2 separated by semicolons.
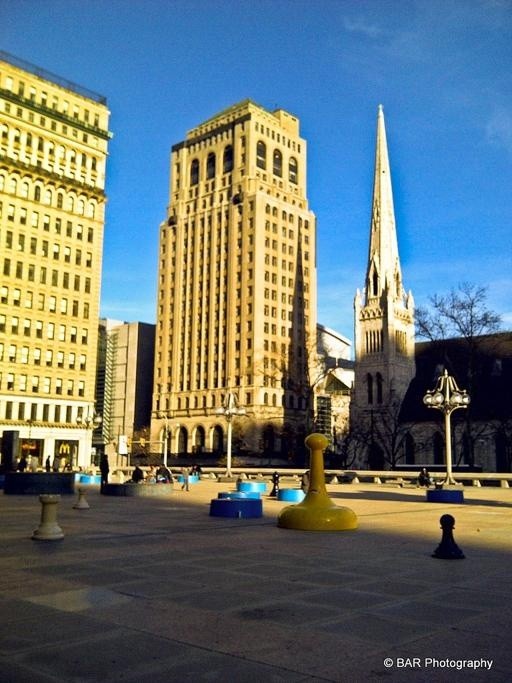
422;369;473;489
214;389;246;482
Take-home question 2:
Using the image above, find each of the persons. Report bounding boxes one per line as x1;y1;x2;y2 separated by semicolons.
100;454;109;486
16;456;91;473
419;467;431;488
132;460;202;491
